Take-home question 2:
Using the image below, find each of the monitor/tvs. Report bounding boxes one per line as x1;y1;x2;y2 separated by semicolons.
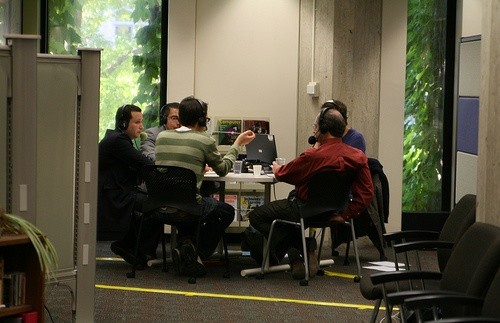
245;135;277;163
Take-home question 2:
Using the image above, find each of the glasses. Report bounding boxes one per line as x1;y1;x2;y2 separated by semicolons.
206;118;210;122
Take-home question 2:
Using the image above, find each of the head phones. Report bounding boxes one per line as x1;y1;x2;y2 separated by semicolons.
119;104;128;130
195;99;207;126
319;107;332;133
160;105;167;124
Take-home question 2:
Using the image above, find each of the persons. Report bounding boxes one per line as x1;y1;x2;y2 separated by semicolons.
321;98;366;152
155;96;255;276
98;104;209;268
248;106;374;278
142;102;180;161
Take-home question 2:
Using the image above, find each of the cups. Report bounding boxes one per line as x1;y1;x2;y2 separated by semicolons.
253;165;262;176
233;161;242;174
276;158;286;166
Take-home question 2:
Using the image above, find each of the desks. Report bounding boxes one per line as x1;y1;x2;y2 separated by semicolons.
202;172;275;265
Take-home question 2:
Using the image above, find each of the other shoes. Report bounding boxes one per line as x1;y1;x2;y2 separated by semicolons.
306;237;318;278
111;240;151;270
170;240;208;277
288;248;305;280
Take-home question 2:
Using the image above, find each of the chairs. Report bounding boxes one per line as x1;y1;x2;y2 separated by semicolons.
97;157;500;323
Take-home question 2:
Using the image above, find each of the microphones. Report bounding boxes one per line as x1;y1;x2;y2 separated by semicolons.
308;129;320;145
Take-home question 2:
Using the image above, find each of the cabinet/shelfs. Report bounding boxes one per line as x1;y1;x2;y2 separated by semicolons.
0;33;103;323
